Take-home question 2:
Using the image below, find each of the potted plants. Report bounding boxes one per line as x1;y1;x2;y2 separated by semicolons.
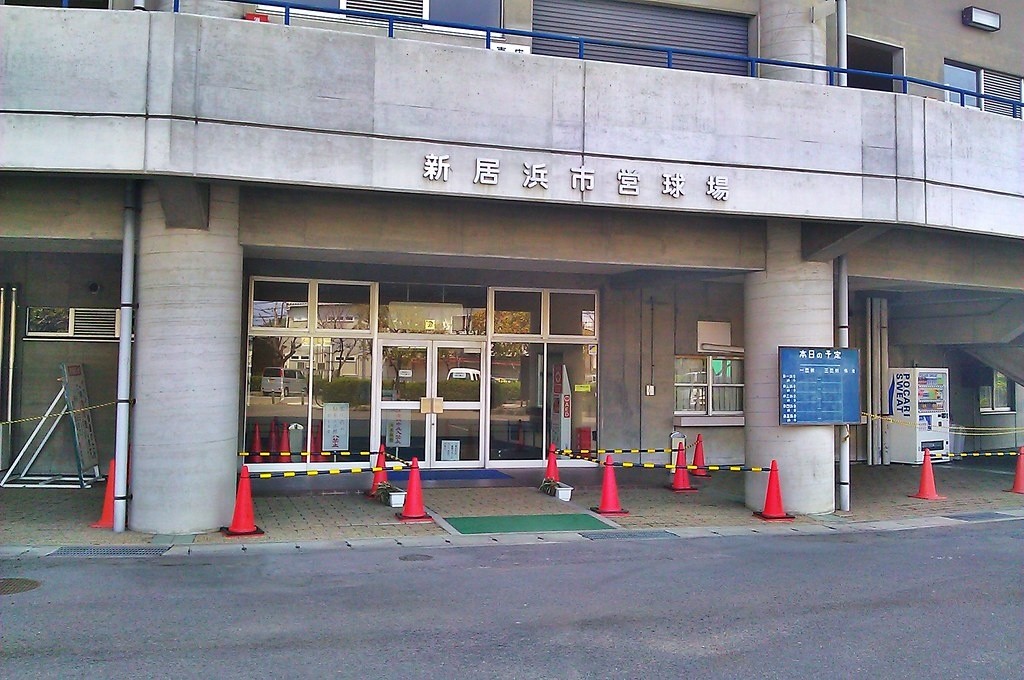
537;478;574;500
372;478;407;507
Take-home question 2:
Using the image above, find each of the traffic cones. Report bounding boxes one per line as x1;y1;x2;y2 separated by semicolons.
220;466;265;537
249;416;291;463
88;459;115;528
1002;446;1024;494
545;443;561;483
753;460;796;522
590;455;631;516
393;457;434;524
690;433;712;479
302;420;328;462
664;442;698;492
907;448;948;500
368;444;389;499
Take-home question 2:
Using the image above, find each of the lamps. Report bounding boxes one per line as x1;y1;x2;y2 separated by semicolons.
961;6;1002;32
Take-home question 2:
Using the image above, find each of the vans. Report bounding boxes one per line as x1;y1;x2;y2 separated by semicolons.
260;367;308;397
446;367;480;381
